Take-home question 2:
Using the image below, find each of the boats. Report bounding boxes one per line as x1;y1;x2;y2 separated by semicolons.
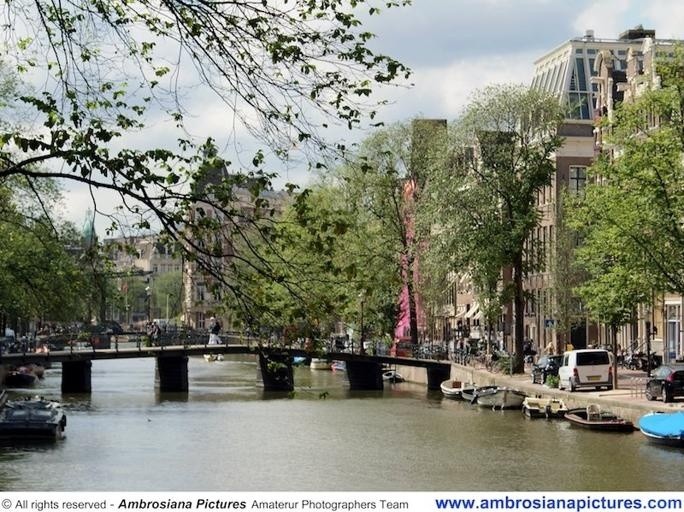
564;405;630;429
0;396;65;440
293;356;347;371
382;370;404;382
6;363;45;386
522;397;568;418
641;412;684;444
440;377;528;407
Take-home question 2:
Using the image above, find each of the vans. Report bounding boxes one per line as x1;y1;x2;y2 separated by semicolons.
557;349;613;391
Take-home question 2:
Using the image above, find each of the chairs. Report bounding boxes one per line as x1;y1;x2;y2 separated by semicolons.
587;403;602;421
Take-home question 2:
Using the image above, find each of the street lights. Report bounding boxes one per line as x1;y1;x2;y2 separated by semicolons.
145;285;152;322
358;292;365;354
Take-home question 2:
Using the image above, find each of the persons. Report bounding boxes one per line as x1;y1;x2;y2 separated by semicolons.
524;335;626;369
208;316;222;345
4;324;15;343
450;331;501;361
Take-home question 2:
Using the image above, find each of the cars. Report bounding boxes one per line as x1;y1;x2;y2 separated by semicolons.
533;356;560;385
391;338;413;356
645;364;684;402
413;344;447;359
